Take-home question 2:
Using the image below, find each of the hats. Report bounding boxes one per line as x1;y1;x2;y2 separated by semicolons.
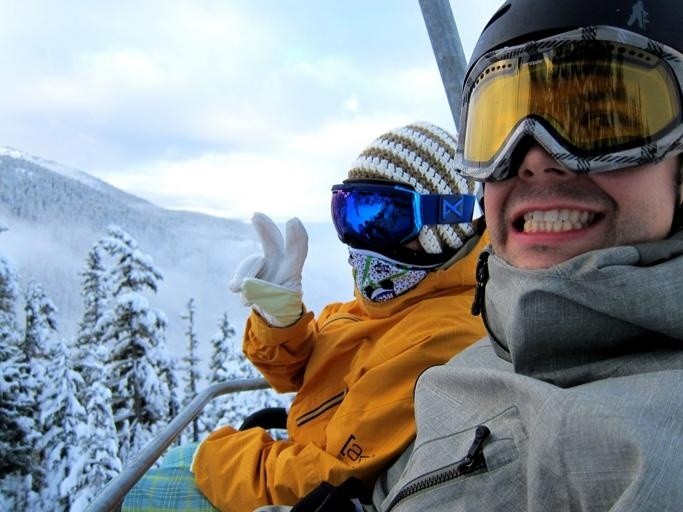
348;121;484;254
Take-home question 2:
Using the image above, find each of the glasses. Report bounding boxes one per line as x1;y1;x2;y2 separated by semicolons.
332;178;484;247
452;25;683;182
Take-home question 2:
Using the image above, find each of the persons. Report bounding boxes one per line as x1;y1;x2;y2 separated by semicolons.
188;119;493;512
249;1;683;512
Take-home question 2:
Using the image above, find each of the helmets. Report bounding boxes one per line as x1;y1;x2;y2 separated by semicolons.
461;0;683;89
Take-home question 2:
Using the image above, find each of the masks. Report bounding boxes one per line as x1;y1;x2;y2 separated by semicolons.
348;245;435;303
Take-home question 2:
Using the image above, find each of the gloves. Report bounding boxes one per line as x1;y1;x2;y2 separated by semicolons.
228;211;308;328
